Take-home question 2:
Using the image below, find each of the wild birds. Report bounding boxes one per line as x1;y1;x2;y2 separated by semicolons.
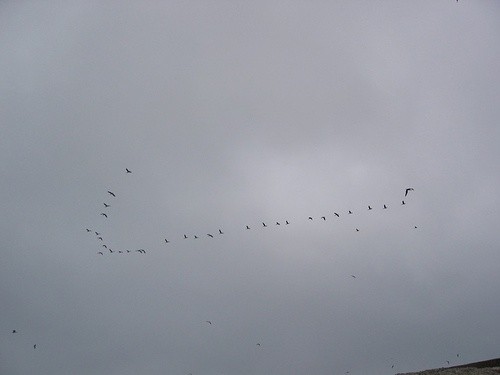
34;344;36;349
12;330;15;333
85;168;418;256
